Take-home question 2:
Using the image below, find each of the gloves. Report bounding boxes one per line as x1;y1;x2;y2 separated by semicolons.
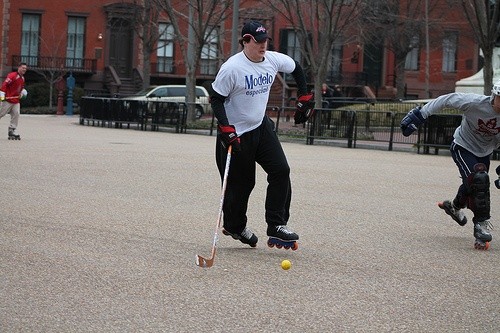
1;95;6;101
293;93;315;123
217;125;241;157
20;95;27;100
400;106;424;137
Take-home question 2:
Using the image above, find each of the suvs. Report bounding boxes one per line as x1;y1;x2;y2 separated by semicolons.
121;84;211;119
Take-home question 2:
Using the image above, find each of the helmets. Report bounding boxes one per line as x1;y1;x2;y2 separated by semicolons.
491;79;500;96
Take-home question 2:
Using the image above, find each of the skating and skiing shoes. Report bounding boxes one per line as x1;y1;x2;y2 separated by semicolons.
438;200;467;226
473;216;495;250
8;128;20;140
267;225;299;250
222;225;258;247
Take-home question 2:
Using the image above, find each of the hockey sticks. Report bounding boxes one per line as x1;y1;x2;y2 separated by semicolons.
194;144;232;268
0;96;20;99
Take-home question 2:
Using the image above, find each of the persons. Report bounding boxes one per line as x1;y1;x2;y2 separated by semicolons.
209;23;312;250
401;80;500;251
292;89;315;128
322;84;334;97
0;63;28;140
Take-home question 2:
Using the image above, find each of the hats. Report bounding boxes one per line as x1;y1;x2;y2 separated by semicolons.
242;22;272;43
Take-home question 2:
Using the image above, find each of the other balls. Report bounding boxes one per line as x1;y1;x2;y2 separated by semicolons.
281;260;291;270
